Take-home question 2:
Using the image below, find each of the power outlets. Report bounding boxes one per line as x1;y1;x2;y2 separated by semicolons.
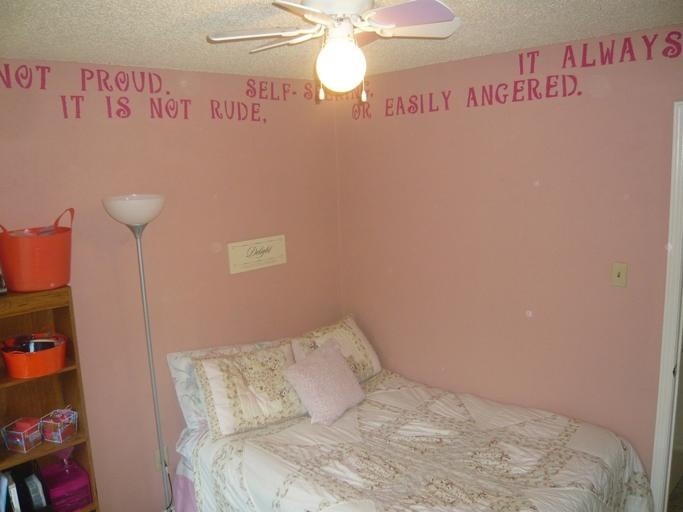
152;445;169;472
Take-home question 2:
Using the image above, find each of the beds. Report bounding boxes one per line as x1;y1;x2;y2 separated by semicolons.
173;370;654;512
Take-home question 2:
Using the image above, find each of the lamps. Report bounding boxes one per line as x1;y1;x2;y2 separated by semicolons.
315;21;367;102
101;193;174;512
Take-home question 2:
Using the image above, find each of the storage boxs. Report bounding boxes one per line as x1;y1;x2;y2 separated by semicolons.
40;457;92;511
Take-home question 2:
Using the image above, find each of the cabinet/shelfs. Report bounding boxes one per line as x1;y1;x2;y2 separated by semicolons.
0;286;100;512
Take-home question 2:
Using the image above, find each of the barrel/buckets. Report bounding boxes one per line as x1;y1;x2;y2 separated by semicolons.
0;208;74;294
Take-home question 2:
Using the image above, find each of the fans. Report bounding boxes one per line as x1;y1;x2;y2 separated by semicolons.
207;0;460;53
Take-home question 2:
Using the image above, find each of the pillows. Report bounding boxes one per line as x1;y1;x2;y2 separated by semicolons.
165;313;382;440
